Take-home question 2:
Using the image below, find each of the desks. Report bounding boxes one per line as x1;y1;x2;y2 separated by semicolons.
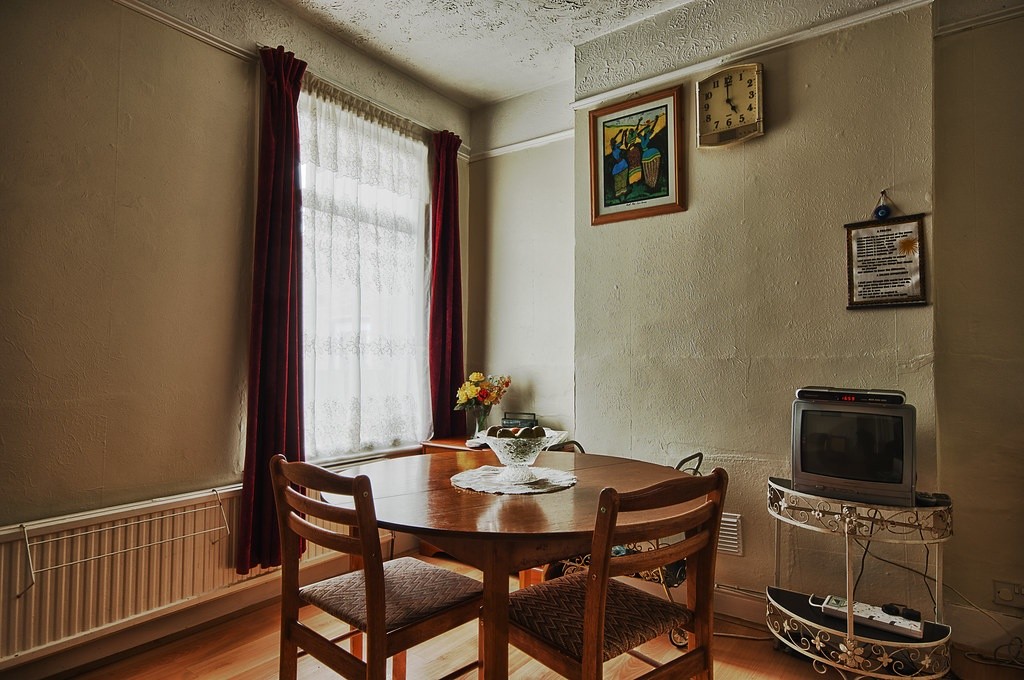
316;449;707;680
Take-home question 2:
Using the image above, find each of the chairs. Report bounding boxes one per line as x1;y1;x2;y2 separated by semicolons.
476;467;730;680
268;453;484;680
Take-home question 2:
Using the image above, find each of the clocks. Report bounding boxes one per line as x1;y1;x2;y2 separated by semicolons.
693;61;765;149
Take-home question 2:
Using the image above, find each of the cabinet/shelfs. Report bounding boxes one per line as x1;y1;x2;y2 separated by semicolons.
766;476;956;679
419;433;574;589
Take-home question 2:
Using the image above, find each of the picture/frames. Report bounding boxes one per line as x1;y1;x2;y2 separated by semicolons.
588;83;687;226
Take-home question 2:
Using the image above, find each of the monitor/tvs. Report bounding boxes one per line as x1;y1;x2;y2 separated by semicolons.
789;399;916;509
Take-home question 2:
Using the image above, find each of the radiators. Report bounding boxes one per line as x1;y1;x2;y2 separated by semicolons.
1;452;396;668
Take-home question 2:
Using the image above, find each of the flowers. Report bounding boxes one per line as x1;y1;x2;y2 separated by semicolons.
454;371;513;424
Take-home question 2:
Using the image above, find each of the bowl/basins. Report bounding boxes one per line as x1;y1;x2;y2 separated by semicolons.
477;426;556;465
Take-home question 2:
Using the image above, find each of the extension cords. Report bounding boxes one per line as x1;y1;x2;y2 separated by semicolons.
822;595;924;637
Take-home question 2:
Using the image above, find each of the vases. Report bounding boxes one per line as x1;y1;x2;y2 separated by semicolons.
469;415;487;438
